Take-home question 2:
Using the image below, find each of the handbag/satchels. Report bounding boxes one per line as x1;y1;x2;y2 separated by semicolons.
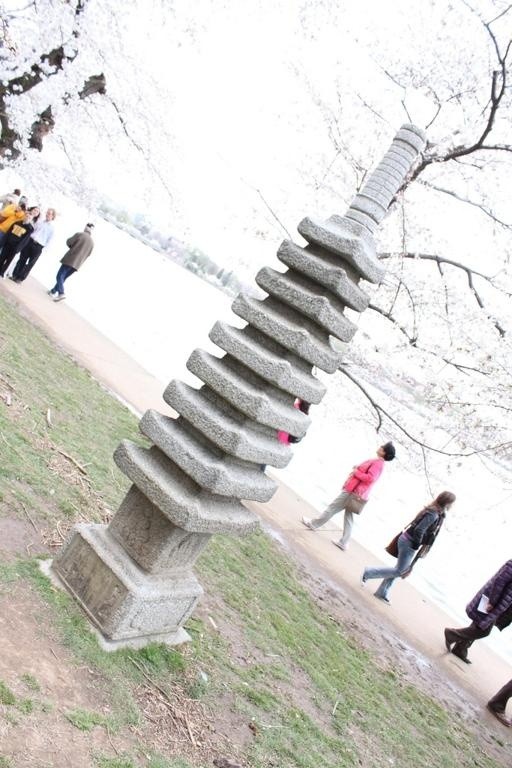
385;531;402;558
344;491;365;515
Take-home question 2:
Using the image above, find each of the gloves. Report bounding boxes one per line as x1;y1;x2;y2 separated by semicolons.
426;533;434;545
411;540;420;550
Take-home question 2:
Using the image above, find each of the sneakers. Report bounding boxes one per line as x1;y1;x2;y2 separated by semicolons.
374;592;391;605
303;516;317;531
0;271;22;284
47;290;65;302
361;567;366;587
332;539;346;550
488;705;511;727
446;639;471;663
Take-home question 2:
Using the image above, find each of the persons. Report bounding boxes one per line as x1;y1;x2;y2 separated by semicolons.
441;556;510;668
46;220;97;302
359;490;458;607
300;437;396;549
276;398;310;447
0;186;57;285
487;678;512;731
